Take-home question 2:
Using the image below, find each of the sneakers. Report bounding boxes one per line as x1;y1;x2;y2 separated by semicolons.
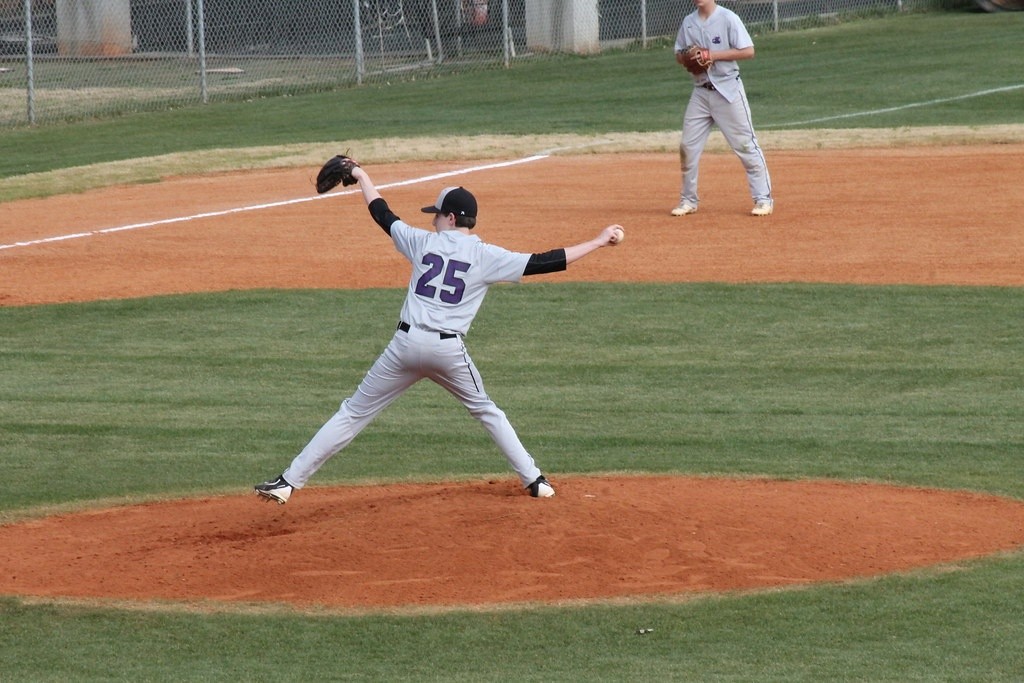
254;475;294;505
751;203;773;216
529;475;555;498
671;204;697;216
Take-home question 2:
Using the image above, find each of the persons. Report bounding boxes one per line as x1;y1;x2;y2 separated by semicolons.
670;0;774;216
255;155;625;506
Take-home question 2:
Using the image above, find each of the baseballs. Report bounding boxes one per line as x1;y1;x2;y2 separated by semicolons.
614;229;624;243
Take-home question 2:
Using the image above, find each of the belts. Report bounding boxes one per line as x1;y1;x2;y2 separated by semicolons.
398;321;456;339
703;83;715;90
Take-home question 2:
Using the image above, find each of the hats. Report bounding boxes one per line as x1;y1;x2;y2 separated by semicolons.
421;186;478;217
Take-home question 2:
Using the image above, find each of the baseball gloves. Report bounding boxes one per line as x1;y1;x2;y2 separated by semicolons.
683;45;712;75
315;153;360;194
